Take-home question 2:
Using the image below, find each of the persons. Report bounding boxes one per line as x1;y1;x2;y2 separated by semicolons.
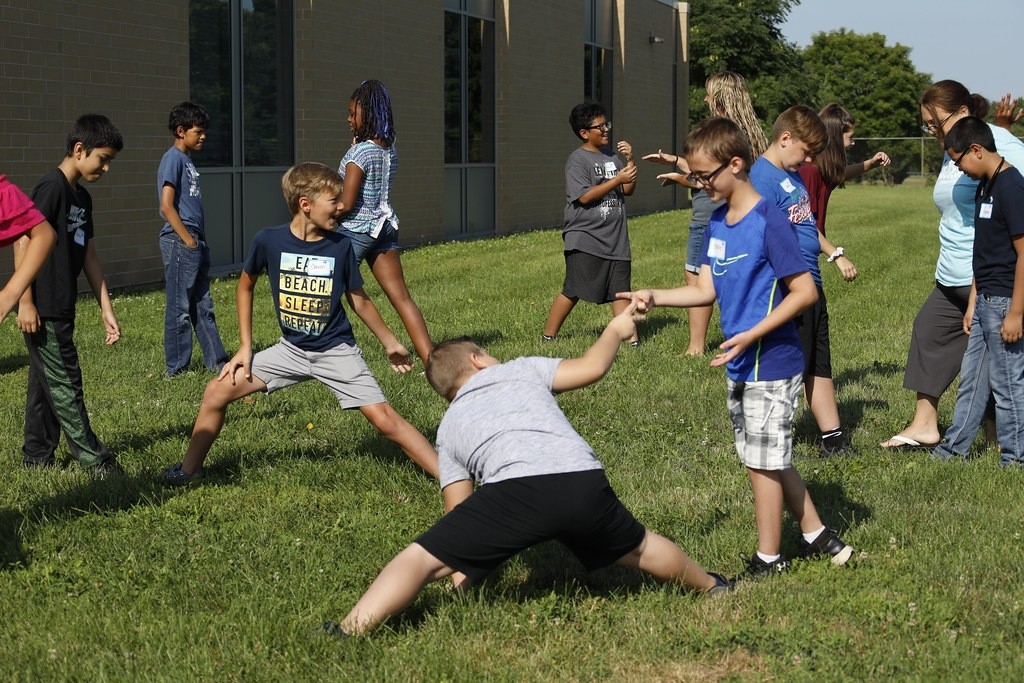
0;171;57;328
155;101;230;381
878;79;1024;474
154;159;440;486
13;112;125;481
318;72;891;649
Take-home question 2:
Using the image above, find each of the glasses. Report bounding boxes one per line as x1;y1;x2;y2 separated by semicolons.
921;104;969;132
954;145;982;168
686;155;737;185
577;121;613;132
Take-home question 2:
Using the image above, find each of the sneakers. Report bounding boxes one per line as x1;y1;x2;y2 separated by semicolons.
786;525;855;566
729;552;792;584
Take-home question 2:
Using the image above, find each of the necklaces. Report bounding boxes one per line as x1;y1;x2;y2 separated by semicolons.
979;157;1004;201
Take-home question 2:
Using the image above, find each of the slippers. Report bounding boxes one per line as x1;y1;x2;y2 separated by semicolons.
880;436;942;453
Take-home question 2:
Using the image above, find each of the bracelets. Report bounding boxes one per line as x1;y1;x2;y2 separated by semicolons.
674;155;678;165
826;246;844;262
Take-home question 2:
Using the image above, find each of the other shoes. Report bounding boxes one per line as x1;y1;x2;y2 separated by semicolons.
819;440;861;459
160;462;205;485
